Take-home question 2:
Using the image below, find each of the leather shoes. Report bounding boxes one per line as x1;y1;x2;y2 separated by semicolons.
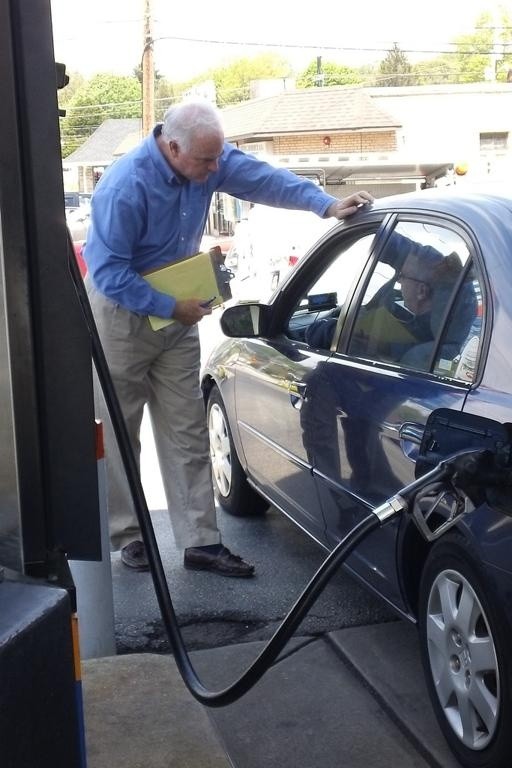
185;548;255;577
122;542;150;572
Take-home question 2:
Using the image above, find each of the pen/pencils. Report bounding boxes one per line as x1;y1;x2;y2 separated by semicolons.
201;296;216;308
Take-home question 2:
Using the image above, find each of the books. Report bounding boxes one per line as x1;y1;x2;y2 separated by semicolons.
139;249;223;334
349;304;419;349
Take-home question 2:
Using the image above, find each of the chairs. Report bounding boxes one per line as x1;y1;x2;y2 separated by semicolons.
399;271;462;371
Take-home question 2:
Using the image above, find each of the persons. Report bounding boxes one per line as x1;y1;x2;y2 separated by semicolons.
77;100;378;581
298;229;480;571
350;238;449;363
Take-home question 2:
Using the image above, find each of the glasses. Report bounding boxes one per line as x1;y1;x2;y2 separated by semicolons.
397;271;423;283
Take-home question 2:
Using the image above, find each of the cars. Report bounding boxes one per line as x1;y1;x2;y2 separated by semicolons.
190;181;509;768
